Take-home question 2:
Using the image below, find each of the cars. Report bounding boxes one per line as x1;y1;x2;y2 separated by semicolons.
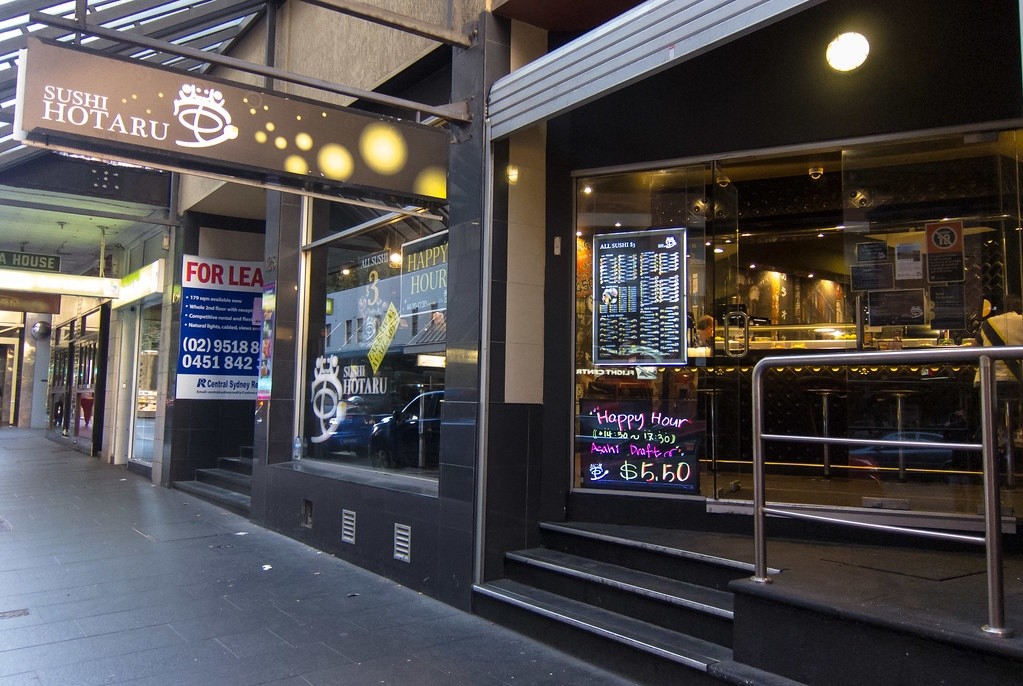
370;391;444;470
317;396;394;456
845;431;1005;483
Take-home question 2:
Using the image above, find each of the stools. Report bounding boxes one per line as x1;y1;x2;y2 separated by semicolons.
996;394;1020;489
878;377;922;482
798;376;862;482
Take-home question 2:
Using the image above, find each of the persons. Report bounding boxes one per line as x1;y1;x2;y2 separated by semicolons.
53;392;63;425
82;392;95;426
971;295;1023;484
698;315;717;340
